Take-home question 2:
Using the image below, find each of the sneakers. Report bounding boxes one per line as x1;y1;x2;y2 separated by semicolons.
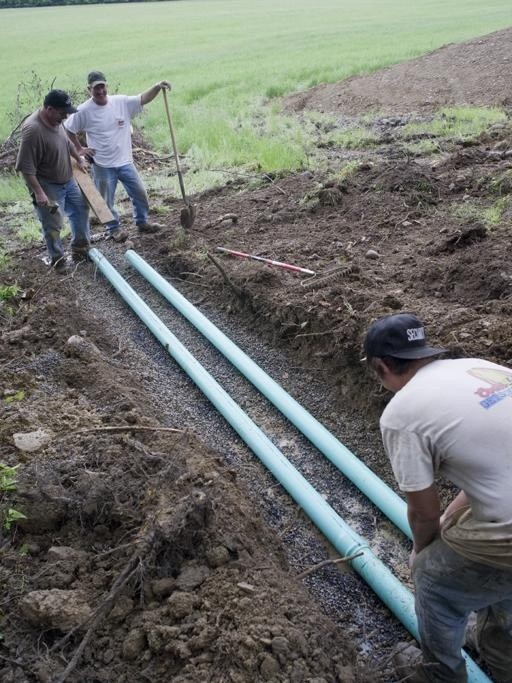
389;642;468;683
465;610;511;681
51;221;161;275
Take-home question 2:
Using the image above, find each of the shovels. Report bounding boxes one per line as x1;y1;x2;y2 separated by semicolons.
162;84;196;228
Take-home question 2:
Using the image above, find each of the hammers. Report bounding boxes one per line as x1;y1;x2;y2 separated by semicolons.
31;193;59;215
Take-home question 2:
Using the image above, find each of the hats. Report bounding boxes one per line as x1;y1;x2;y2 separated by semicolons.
364;313;451;359
44;89;79;113
86;70;106;88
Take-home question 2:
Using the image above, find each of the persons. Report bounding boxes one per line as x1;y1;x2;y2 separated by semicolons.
355;311;512;683
63;69;172;242
15;87;96;274
